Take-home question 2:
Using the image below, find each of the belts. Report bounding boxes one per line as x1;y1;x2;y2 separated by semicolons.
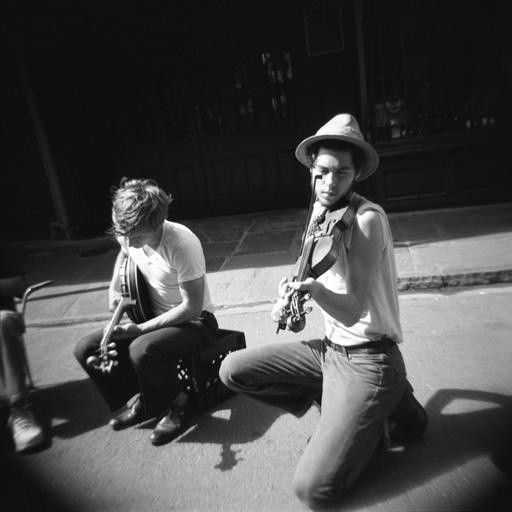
325;336;395;354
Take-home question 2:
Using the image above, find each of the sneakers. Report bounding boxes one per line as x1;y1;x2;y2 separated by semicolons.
391;380;428;433
8;411;43;452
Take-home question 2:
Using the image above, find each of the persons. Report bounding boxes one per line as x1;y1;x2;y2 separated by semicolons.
74;177;206;444
1;227;47;453
219;113;428;512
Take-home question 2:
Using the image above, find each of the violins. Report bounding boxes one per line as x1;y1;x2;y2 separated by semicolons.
287;202;355;332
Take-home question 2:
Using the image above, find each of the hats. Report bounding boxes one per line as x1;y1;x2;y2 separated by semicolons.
295;113;379;181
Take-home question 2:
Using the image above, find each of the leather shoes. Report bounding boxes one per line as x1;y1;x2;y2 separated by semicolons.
150;411;184;444
109;398;142;430
